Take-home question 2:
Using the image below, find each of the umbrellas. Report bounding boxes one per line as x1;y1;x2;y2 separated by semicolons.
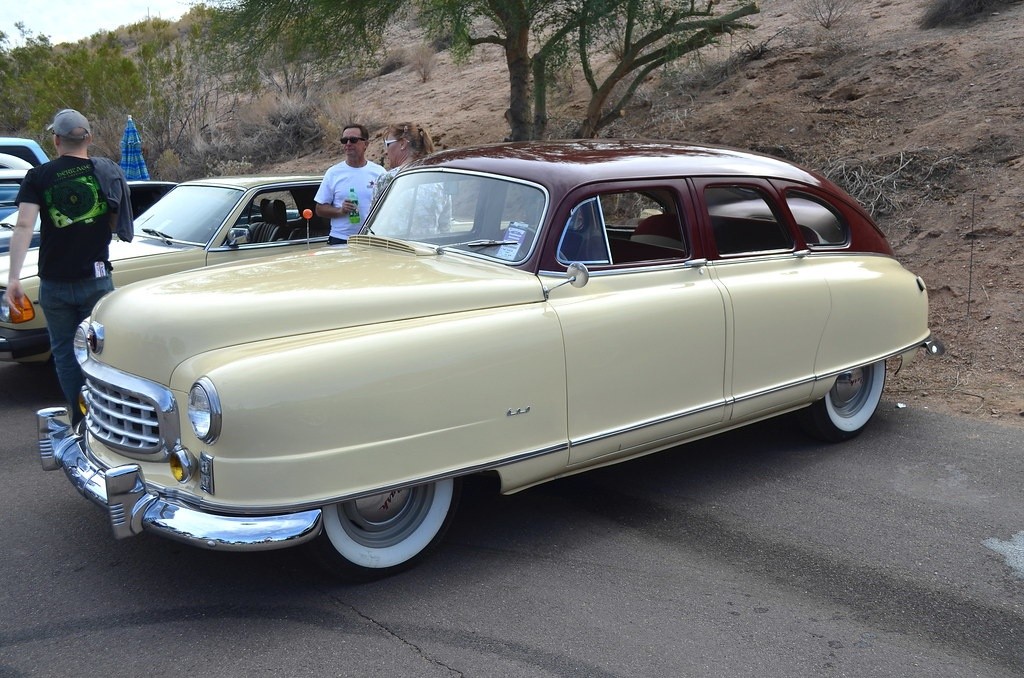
119;115;150;181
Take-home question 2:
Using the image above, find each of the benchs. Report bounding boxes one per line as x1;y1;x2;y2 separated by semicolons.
634;214;821;254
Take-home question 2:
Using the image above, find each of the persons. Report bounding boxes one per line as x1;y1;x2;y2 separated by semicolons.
3;109;135;426
314;123;388;246
366;122;456;235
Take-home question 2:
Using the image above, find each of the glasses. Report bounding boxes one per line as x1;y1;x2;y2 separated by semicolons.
339;136;365;145
51;129;59;138
384;139;410;148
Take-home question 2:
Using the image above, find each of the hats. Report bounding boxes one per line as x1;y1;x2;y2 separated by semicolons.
46;109;90;140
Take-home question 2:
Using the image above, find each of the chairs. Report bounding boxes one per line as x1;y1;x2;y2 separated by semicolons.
245;198;287;243
288;208;331;240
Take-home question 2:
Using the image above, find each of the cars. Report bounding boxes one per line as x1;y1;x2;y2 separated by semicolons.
0;138;520;359
37;139;946;584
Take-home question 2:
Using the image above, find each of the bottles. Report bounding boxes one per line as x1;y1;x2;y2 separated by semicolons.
348;188;360;223
